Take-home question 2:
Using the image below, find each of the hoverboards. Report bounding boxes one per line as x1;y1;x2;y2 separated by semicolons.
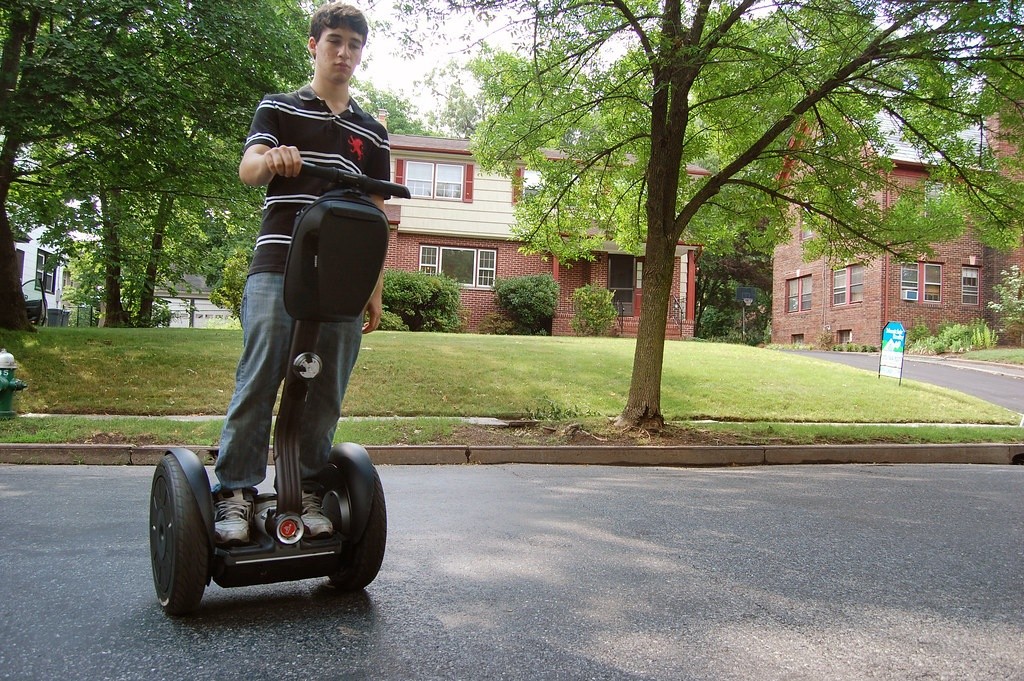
148;156;413;616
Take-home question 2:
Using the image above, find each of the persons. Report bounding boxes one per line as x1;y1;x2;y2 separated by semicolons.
211;2;391;546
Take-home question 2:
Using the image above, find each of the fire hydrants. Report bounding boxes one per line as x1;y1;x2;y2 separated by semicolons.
0;347;29;418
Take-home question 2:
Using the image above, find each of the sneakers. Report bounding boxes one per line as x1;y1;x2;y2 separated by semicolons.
215;489;255;546
301;491;333;539
